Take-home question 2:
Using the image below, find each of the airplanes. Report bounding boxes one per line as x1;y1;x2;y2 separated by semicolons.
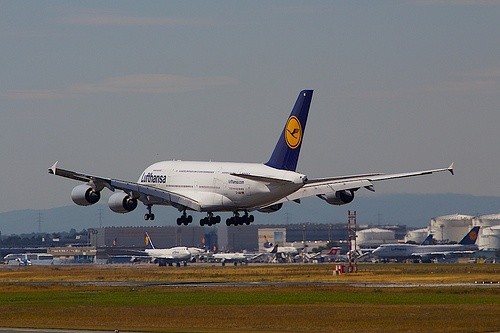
209;250;271;268
264;228;499;267
47;90;456;227
108;231;206;266
3;253;32;265
